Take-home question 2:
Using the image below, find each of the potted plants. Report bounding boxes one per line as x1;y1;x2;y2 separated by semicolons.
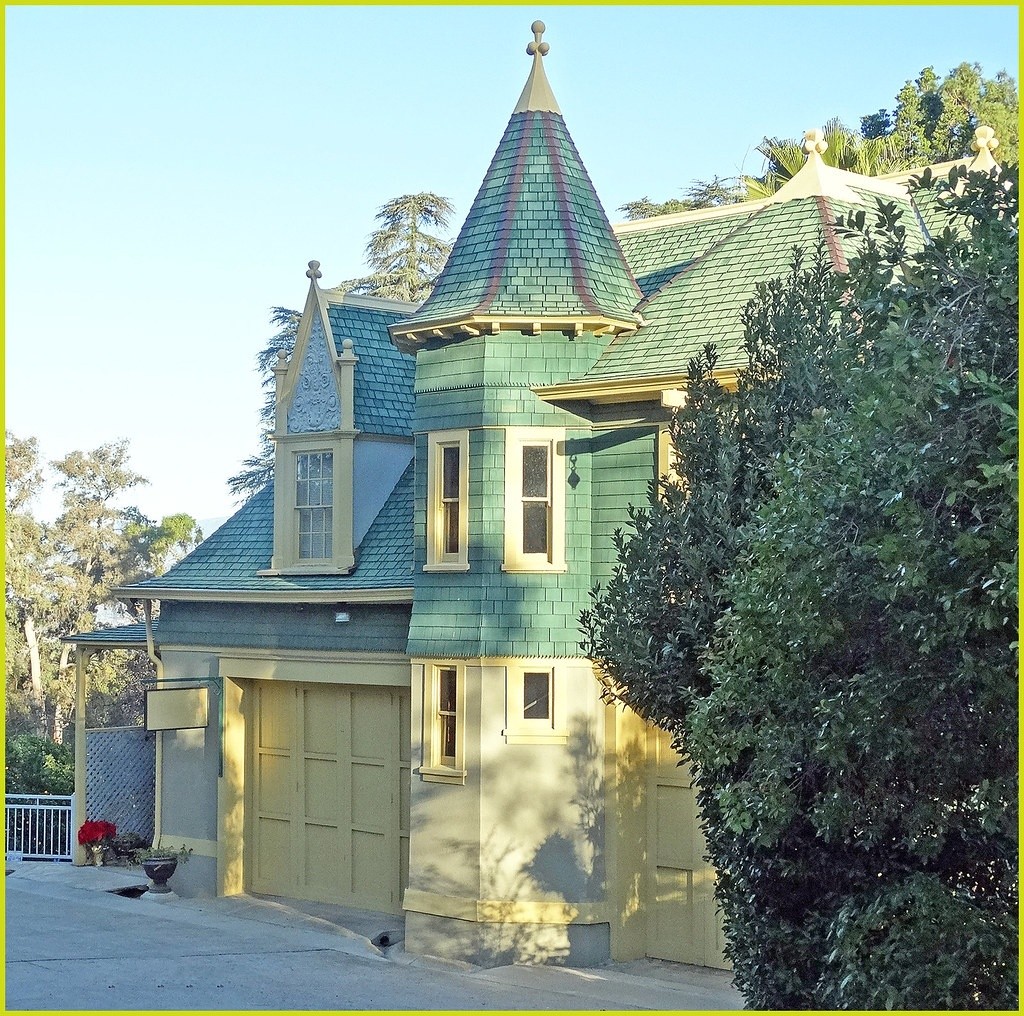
126;843;194;894
110;832;142;855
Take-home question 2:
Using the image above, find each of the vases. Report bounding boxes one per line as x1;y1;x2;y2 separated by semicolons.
90;842;105;867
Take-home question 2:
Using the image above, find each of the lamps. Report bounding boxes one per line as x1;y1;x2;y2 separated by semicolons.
334;606;353;623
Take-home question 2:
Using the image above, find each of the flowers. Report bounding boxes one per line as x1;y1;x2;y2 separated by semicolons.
78;817;116;845
